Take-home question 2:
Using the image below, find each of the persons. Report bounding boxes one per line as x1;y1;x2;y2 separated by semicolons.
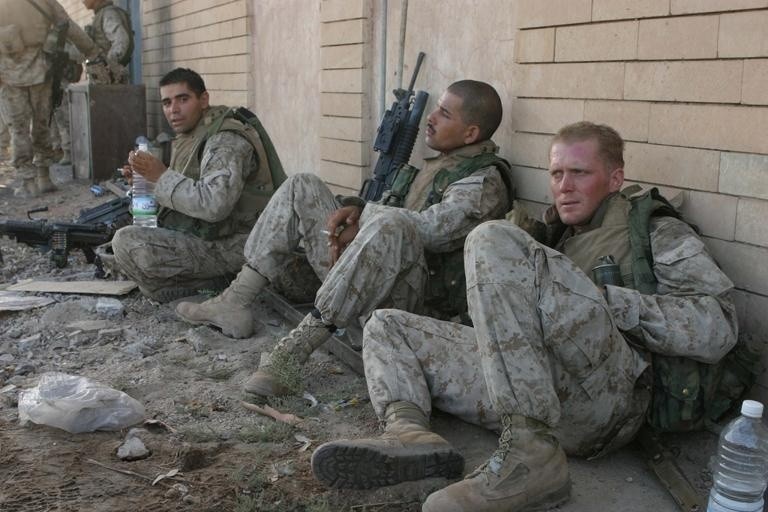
110;67;289;304
308;120;741;512
0;0;132;198
174;78;517;406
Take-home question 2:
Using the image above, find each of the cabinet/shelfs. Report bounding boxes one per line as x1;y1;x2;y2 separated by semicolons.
65;82;146;183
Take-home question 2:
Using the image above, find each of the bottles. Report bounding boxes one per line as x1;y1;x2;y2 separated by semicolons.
703;398;767;510
131;136;159;228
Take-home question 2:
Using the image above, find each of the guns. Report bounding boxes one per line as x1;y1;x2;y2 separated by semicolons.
359;51;429;200
49;51;69;128
1;197;133;267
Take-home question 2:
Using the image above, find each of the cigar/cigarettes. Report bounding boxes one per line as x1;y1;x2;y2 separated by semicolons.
320;229;339;238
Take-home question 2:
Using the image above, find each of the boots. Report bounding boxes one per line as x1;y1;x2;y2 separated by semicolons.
174;262;272;340
36;166;59;194
241;308;339;406
309;398;466;491
59;150;72;166
14;177;40;199
420;410;574;512
